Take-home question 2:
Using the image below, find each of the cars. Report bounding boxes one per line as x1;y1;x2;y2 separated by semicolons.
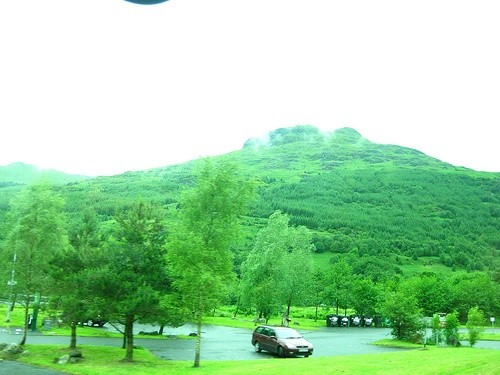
251;325;314;358
61;310;110;327
